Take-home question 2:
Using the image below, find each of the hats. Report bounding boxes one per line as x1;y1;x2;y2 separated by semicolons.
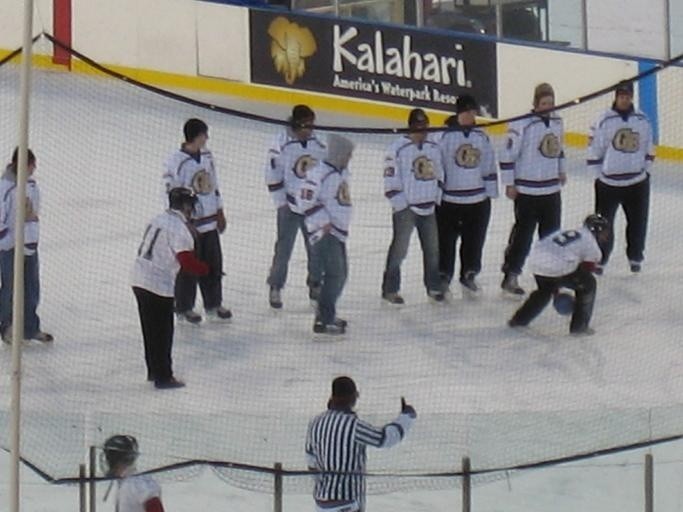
534;83;556;103
616;82;636;96
409;107;430;126
182;117;208;139
455;94;478;111
586;215;607;233
170;188;199;211
292;105;314;124
332;376;355;394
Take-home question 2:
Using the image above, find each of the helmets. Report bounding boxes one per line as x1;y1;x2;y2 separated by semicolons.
97;434;140;462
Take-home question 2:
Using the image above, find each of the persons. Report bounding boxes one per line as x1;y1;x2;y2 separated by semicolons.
299;134;354;334
499;82;567;295
381;109;445;307
101;434;163;512
265;104;329;309
163;118;232;323
1;147;56;346
586;81;655;273
508;213;611;337
131;186;217;389
305;376;416;512
439;94;500;298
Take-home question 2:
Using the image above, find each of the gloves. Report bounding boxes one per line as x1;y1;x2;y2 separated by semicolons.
209;263;228;281
401;397;418;421
217;212;226;233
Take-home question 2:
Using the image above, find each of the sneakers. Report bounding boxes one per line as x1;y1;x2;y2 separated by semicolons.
564;327;597;337
427;288;443;303
315;321;342;333
156;379;187;387
630;261;640;272
208;307;233;319
177;309;201;321
307;287;323;304
460;275;484;295
268;286;283;311
500;273;525;295
382;292;405;308
512;321;533;332
34;332;55;342
